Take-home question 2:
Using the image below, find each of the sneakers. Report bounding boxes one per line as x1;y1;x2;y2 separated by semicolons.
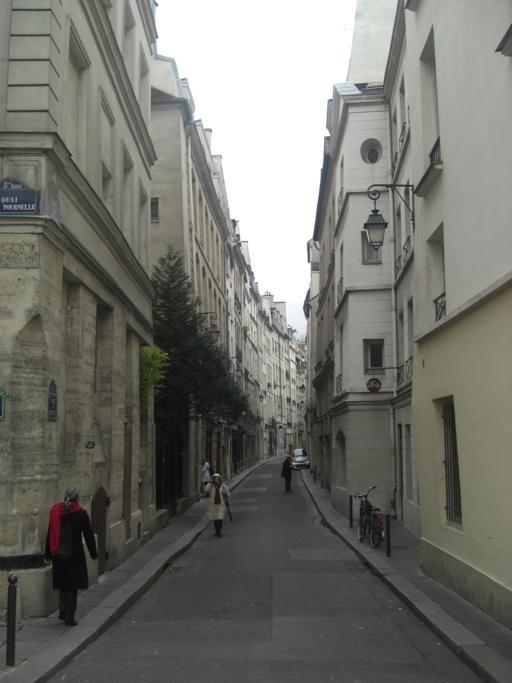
216;531;221;537
58;611;65;620
65;620;79;627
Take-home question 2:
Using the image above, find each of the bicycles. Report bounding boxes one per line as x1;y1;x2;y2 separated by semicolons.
354;484;386;549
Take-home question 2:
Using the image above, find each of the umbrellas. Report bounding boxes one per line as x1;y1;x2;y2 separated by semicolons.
223;492;232;523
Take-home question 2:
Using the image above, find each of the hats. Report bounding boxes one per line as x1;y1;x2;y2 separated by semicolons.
212;473;223;481
64;486;80;500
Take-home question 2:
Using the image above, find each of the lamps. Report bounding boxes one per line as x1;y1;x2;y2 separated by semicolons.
363;183;414;251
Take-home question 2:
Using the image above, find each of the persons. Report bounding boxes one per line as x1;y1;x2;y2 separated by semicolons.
44;486;98;625
202;459;212;496
280;453;295;494
204;471;229;539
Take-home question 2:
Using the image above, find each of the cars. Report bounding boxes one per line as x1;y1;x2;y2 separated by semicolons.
291;448;310;470
